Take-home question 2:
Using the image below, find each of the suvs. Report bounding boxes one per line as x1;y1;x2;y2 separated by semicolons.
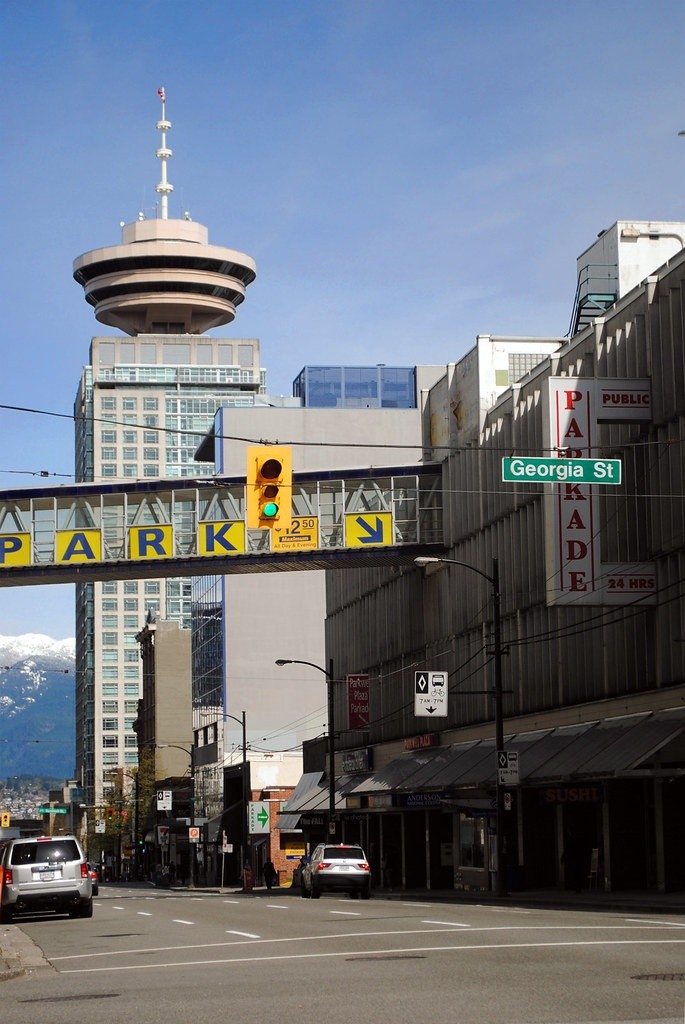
0;832;92;923
302;843;370;899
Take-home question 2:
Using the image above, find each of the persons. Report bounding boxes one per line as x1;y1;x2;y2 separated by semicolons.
264;857;276;890
243;862;255;892
169;860;175;886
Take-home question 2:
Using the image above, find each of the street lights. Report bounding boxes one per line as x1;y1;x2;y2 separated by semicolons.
111;773;139;881
200;711;247;868
275;658;336;845
158;743;196;887
413;556;521;897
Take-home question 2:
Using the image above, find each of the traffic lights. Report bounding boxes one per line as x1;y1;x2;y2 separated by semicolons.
106;808;114;825
247;446;292;529
1;813;10;827
139;835;143;845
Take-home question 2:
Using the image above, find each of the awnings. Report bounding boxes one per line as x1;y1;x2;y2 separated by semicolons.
276;771;375;829
342;707;684;796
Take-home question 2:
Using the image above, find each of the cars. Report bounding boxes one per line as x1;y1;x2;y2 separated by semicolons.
87;862;98;896
